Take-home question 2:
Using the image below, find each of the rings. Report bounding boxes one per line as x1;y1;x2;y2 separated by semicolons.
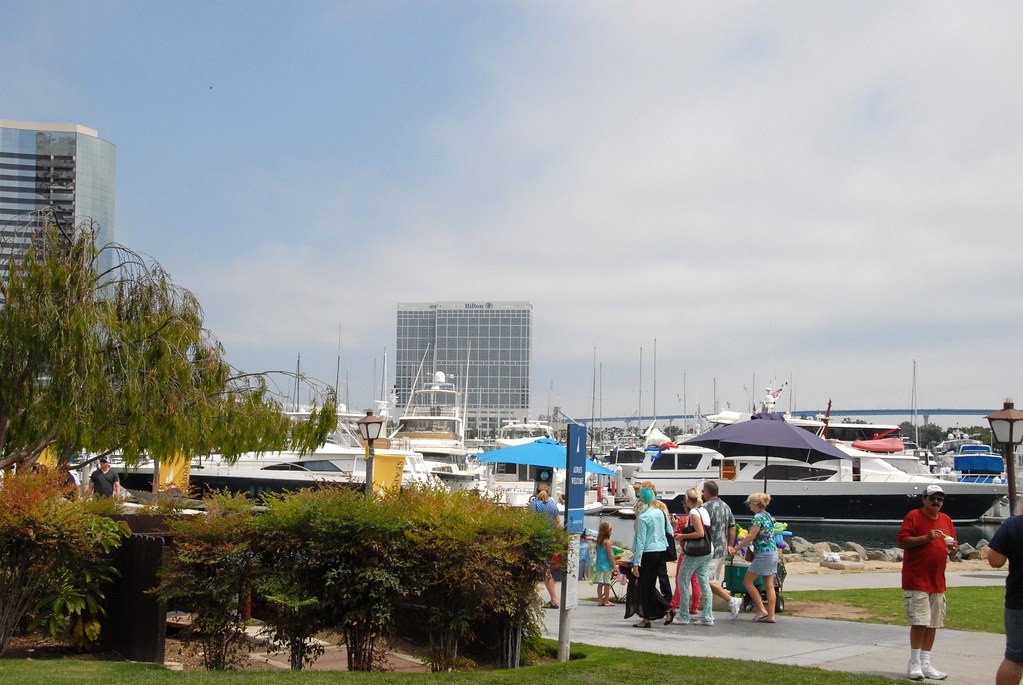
952;547;956;549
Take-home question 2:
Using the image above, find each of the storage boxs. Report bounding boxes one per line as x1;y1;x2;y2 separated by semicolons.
725;562;766;593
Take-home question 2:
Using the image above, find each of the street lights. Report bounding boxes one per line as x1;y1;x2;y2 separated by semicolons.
983;399;1023;516
357;410;387;496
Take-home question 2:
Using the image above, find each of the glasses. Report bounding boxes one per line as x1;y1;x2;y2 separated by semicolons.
925;495;944;502
99;459;106;463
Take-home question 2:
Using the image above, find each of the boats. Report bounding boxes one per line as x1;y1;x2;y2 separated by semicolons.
283;336;1005;484
623;384;1010;525
386;341;472;463
80;438;445;498
442;438;616;517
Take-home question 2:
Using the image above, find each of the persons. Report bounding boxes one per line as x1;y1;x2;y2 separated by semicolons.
736;493;779;623
85;457;120;502
528;481;563;609
895;485;961;681
622;481;676;628
669;481;743;627
594;522;617;605
60;461;82;500
984;515;1023;685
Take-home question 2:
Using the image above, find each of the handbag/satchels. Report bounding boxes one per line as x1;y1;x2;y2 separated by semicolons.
661;511;677;562
682;507;712;556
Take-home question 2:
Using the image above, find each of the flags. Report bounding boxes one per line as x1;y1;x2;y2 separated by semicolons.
772;389;782;397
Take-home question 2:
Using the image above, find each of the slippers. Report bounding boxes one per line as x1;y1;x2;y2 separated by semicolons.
597;603;604;606
603;602;616;606
757;619;776;623
752;612;769;621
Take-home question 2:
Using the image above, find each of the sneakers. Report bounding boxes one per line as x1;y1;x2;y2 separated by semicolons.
729;597;743;622
921;665;947;680
692;613;716;622
907;660;924;680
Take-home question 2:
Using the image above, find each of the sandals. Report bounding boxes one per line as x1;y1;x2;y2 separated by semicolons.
541;599;559;608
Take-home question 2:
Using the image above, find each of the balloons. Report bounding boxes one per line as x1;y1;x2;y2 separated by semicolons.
733;520;794;557
643;442;678;462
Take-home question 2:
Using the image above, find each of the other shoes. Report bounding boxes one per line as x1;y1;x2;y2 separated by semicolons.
633;621;652;628
664;609;677;625
671;616;689;625
693;618;714;627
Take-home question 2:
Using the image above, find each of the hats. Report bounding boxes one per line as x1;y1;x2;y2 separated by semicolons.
100;456;111;462
922;484;945;499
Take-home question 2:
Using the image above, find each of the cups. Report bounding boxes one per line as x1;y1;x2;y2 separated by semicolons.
943;538;954;550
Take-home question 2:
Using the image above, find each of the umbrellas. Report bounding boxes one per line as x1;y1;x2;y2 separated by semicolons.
677;412;854;494
475;437;619;481
